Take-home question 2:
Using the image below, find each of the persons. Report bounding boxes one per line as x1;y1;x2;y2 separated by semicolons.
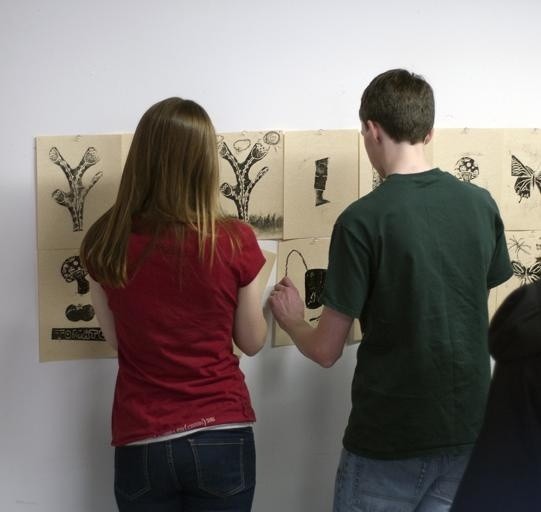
267;68;514;512
80;97;268;511
